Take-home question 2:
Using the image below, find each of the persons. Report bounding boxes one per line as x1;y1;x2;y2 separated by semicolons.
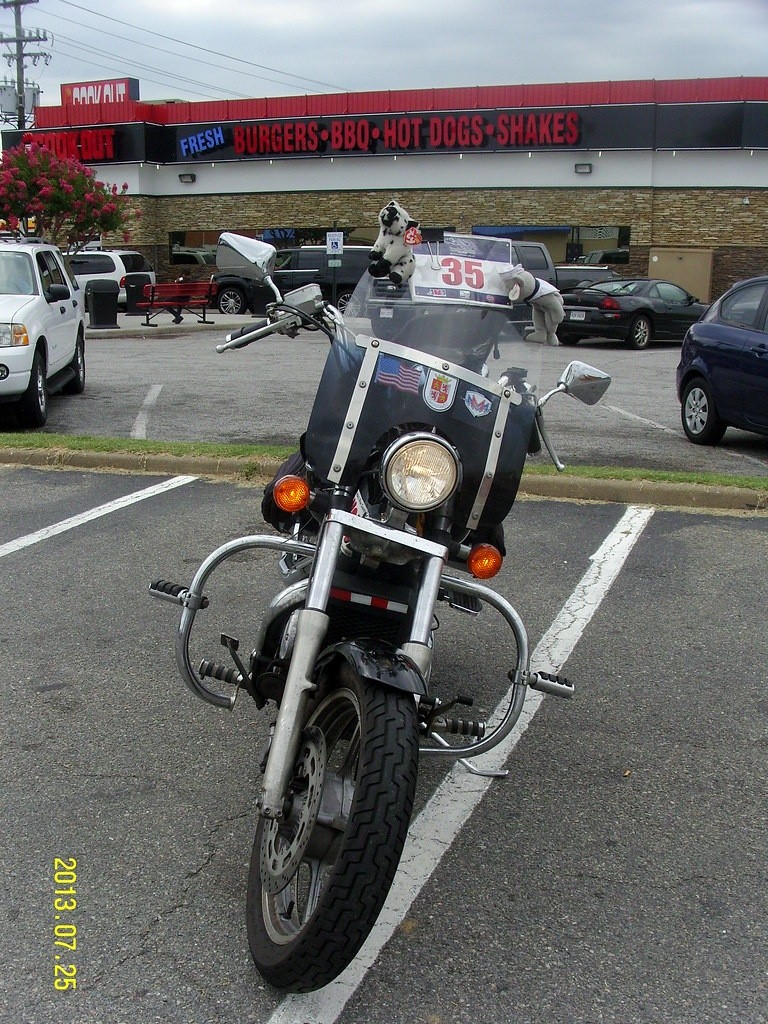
274;256;291;270
163;269;191;324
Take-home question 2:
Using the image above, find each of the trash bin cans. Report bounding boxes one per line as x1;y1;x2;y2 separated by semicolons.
124;274;151;316
86;280;121;329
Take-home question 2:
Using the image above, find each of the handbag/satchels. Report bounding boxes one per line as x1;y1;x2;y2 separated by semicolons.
261;450;315;534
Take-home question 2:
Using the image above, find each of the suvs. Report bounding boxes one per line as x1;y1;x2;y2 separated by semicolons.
62;250;156;311
213;245;376;318
0;236;85;429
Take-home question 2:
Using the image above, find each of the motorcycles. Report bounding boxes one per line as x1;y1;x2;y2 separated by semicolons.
149;233;612;994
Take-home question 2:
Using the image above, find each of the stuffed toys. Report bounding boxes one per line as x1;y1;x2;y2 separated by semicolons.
499;263;564;346
369;201;420;284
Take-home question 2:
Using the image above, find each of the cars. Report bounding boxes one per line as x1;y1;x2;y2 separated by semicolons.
556;277;712;350
677;275;768;446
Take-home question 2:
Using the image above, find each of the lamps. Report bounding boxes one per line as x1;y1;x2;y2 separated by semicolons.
178;174;196;183
574;163;592;173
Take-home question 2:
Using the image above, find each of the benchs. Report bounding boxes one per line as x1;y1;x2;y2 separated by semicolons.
136;281;218;327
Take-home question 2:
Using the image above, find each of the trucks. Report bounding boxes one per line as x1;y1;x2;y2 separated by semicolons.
478;240;614;336
